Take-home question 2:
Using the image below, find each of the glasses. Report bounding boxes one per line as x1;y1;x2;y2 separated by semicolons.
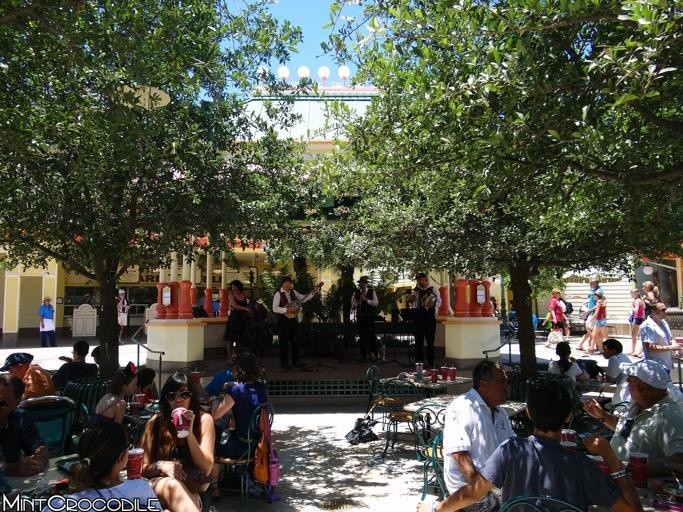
165;391;192;401
0;399;18;407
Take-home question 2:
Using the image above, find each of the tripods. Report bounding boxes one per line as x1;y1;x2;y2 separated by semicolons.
302;289;338;373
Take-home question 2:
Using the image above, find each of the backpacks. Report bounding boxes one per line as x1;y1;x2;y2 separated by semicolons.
561;298;573;314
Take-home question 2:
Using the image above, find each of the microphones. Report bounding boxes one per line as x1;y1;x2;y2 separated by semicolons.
317;282;324;290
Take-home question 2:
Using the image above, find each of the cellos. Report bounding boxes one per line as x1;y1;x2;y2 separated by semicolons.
240;270;274;353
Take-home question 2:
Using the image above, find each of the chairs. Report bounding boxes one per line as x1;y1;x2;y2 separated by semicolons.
497;494;581;512
203;401;275;511
364;365;406;431
15;395;78;463
413;404;455;505
379;379;427;459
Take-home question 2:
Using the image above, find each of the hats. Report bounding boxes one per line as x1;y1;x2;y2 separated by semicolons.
40;297;52;303
414;273;426;280
230;353;265;368
0;352;34;372
357;276;369;283
619;359;669;389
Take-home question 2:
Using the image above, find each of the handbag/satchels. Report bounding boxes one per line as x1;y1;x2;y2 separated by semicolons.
548;324;563;344
254;407;269;483
265;407;279;485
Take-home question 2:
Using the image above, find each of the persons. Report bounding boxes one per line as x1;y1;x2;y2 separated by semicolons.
44;341;98;397
639;302;683;379
601;339;633;382
407;273;442;365
223;280;260;366
549;343;584;384
547;288;568;341
41;421;164;512
1;352;33;397
576;331;591;351
273;276;322;368
37;296;57;346
134;371;215;512
0;373;50;476
584;359;683;485
22;365;56;402
626;289;644;356
212;353;269;497
641;281;659;320
136;367;159;399
204;352;258;397
116;290;130;344
584;291;609;355
95;369;137;424
90;345;122;394
585;276;606;353
444;360;518;511
543;292;574;347
351;277;381;362
416;374;644;511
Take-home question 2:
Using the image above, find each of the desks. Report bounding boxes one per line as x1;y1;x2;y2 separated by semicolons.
143;319;229;374
424;315;501;370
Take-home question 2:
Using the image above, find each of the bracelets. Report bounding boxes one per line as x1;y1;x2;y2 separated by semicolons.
610;467;630;479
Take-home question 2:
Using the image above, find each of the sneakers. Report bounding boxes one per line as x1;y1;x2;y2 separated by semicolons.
118;341;126;344
545;343;550;347
41;344;48;347
593;348;604;354
576;347;586;351
51;344;57;347
625;352;635;355
583;349;593;356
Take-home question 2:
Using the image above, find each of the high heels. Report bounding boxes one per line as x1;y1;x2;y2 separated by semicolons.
212;489;221;502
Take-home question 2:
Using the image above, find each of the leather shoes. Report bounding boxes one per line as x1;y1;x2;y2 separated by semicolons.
292;362;306;367
281;364;292;369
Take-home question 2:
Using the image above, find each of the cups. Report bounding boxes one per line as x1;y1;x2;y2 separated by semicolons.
126;447;144;479
441;366;448;383
560;442;577;452
449;367;456;382
675;337;683;355
171;407;190;439
130;402;141;416
135;393;146;407
430;369;439;384
560;429;576;442
629;452;650;490
191;371;201;387
415;363;424;385
